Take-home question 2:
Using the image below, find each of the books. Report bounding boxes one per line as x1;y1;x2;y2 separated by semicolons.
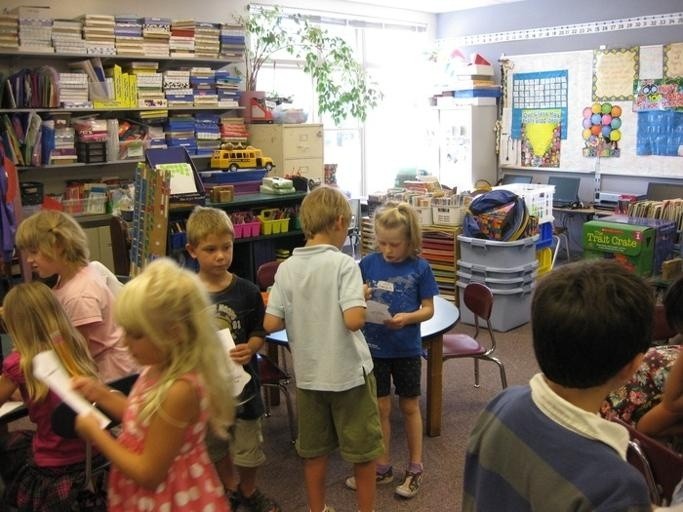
65;183;108;214
617;194;683;232
437;50;500;107
1;11;249;164
368;177;470;208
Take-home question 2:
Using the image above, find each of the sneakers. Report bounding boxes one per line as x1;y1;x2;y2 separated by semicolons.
394;469;424;498
236;480;281;511
344;465;394;492
224;486;241;510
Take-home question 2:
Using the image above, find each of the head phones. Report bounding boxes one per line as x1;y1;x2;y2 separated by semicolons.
568;200;578;209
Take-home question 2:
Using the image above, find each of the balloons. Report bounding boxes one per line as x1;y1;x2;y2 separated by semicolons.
580;102;622;145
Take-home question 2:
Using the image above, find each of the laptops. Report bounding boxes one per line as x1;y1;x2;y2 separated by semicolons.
547;176;580;208
501;174;533;185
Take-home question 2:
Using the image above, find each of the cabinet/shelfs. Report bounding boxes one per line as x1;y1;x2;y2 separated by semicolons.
119;191;311;292
246;122;325;188
438;106;499;192
2;48;246;290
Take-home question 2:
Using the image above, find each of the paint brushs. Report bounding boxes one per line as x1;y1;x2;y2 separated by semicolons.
228;212;244;224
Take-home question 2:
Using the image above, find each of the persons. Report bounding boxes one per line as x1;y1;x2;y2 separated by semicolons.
598;337;683;439
0;282;112;512
345;200;440;497
15;209;147;436
461;259;655;512
70;258;235;512
186;205;283;512
263;185;384;512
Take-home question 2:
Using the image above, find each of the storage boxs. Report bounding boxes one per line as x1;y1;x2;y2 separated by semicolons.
583;221;655;282
366;199;468;229
597;214;677;276
457;231;540;332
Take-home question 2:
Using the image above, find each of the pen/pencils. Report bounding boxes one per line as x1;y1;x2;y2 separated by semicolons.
291;203;300;214
366;279;370;287
169;218;186;232
274;207;291;219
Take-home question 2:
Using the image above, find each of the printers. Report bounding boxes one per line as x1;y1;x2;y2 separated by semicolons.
595;191;620;211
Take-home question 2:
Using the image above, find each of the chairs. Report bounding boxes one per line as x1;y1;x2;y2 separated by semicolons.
50;373;140;512
255;262;295;375
612;418;683;511
651;276;683;346
421;283;509;389
552;226;571;270
244;354;299;443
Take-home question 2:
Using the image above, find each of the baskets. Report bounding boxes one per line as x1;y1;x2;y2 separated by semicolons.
49;193;109;217
430;204;467;227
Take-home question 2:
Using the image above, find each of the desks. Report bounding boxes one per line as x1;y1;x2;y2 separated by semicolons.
1;397;29;434
266;295;461;437
553;206;616;232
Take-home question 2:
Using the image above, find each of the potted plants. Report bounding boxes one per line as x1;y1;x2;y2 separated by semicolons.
230;5;384;124
